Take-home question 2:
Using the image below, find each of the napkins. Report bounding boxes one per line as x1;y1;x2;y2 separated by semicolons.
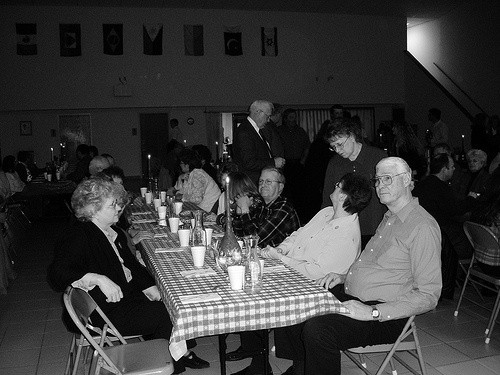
155;247;184;253
264;264;289;273
133;211;151;214
178;292;220;305
153;233;168;237
132;218;156;224
181;268;216;277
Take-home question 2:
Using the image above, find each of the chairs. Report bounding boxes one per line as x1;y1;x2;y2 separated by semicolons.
453;219;500;335
64;285;175;375
344;305;427;375
0;195;32;292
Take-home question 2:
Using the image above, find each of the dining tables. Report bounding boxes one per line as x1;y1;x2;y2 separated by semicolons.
120;190;350;375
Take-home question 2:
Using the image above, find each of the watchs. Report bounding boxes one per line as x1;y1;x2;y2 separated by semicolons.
370;305;379;321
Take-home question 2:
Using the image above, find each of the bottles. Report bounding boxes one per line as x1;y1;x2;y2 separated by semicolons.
152;177;160;200
192;210;206;247
164;195;175;219
243;235;262;295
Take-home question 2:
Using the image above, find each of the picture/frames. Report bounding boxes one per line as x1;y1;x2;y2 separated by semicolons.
20;120;32;136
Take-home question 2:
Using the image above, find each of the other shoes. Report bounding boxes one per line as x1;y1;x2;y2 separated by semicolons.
182;351;210;369
226;347;253;361
280;361;304;375
230;363;273;375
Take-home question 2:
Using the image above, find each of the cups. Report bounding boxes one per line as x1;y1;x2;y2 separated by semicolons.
178;229;190;246
157;206;166;220
140;187;147;197
153;199;161;211
190;218;195;229
44;167;63;181
160;191;166;202
250;259;264;277
173;202;183;215
205;228;213;246
227;265;246;291
191;245;206;266
168;218;179;232
144;193;152;204
237;241;243;251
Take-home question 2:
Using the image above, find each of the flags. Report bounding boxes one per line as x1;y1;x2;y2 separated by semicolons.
184;24;204;56
261;27;278;56
15;23;38;55
103;24;124;55
223;26;243;56
59;24;81;57
143;24;163;56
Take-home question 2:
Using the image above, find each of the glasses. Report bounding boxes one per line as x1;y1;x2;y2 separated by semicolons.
329;134;352;151
334;181;344;193
257;177;281;185
370;172;405;187
262;110;271;120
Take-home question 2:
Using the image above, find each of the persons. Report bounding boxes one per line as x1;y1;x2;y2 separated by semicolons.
65;99;500;305
225;172;372;374
47;177;210;370
0;156;40;224
275;156;442;374
